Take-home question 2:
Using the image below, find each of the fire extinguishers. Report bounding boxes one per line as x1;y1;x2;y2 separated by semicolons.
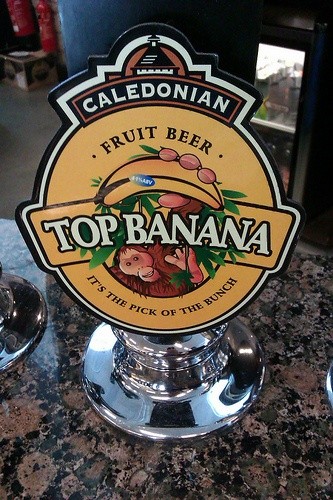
35;0;60;52
6;0;35;36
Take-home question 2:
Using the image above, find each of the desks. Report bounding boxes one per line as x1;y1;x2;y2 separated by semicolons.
0;219;333;500
